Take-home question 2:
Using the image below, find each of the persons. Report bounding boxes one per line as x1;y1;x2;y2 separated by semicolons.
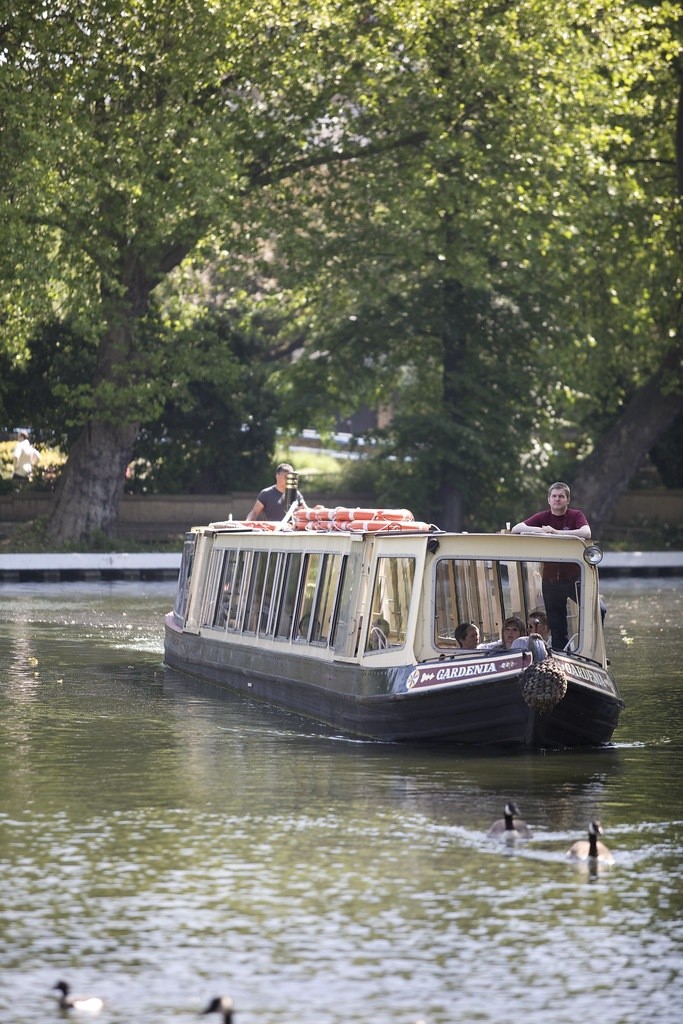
492;617;526;653
526;612;553;648
13;431;40;478
356;616;389;650
299;614;327;641
511;482;611;667
245;463;308;521
453;623;479;650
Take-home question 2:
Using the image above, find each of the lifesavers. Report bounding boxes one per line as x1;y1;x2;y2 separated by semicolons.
292;506;432;532
241;521;276;532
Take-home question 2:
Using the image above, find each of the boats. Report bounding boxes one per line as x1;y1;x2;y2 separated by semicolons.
163;508;626;751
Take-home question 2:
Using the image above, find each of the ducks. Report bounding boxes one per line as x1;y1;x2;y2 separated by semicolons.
47;980;105;1013
564;817;612;865
486;800;532;843
201;998;243;1024
